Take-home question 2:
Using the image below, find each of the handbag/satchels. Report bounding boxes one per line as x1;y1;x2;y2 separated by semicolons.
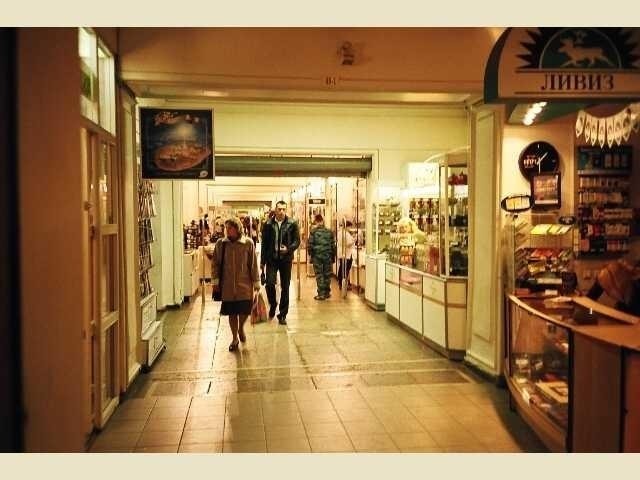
260;267;266;286
211;284;223;302
250;290;269;325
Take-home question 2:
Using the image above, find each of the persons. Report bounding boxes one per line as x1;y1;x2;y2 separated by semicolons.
397;218;428;245
200;213;260;248
336;220;359;291
210;218;261;352
307;214;337;301
262;199;301;326
595;262;638;318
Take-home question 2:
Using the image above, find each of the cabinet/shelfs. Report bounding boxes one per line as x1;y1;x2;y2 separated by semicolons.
574;143;632;259
372;147;468;277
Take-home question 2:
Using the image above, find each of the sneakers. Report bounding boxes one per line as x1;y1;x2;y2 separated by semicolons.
269;302;279;319
276;314;287;326
314;292;333;302
238;329;247;343
229;338;240;351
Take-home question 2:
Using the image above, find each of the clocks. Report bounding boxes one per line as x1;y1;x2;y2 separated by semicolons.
518;141;560;182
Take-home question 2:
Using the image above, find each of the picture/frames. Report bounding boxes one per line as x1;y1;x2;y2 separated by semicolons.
531;171;561;210
